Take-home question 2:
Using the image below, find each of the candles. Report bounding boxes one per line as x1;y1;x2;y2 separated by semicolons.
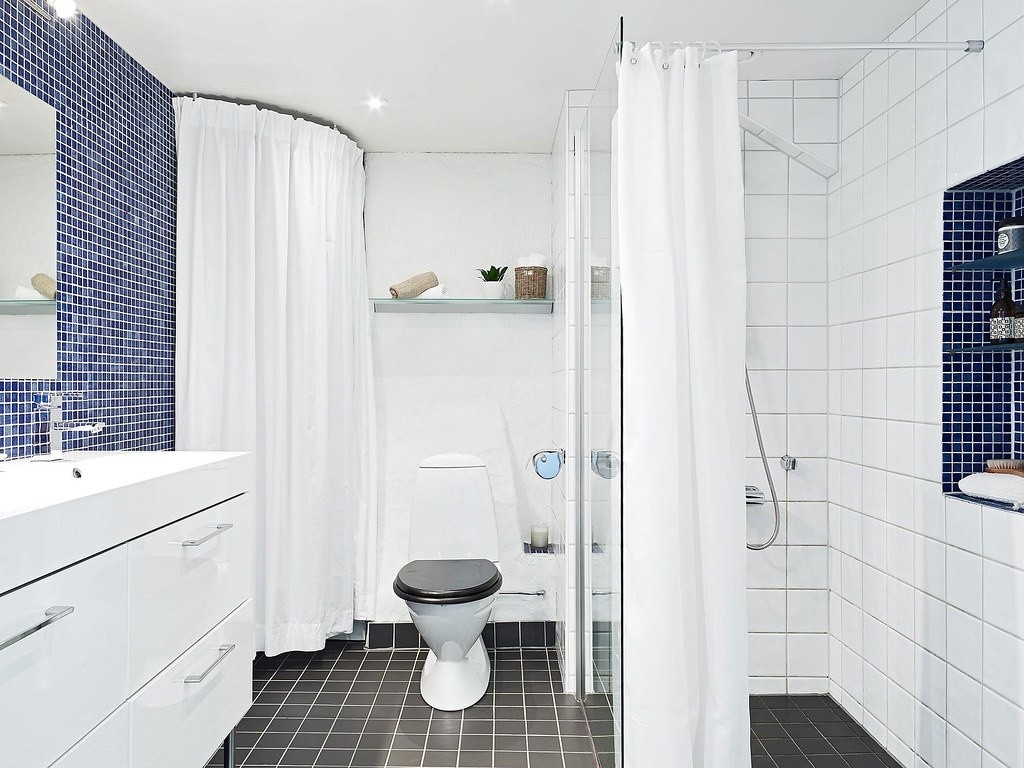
531;524;549;550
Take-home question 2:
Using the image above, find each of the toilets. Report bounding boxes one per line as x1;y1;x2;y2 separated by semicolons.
392;453;504;712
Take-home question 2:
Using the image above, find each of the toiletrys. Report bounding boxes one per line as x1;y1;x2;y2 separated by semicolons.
988;276;1024;344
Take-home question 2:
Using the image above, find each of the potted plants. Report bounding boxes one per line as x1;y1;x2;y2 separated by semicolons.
475;265;509;299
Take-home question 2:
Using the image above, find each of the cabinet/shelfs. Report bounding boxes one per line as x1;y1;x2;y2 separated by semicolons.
947;248;1024;351
0;448;256;768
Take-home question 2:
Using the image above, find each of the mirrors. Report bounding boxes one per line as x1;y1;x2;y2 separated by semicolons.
0;73;59;378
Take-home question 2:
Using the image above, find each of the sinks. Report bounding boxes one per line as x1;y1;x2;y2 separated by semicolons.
0;454;194;517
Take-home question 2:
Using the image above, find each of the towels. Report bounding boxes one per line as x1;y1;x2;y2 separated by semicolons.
14;273;57;301
389;270;449;301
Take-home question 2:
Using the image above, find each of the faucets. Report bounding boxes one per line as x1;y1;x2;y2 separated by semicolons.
30;393;106;463
